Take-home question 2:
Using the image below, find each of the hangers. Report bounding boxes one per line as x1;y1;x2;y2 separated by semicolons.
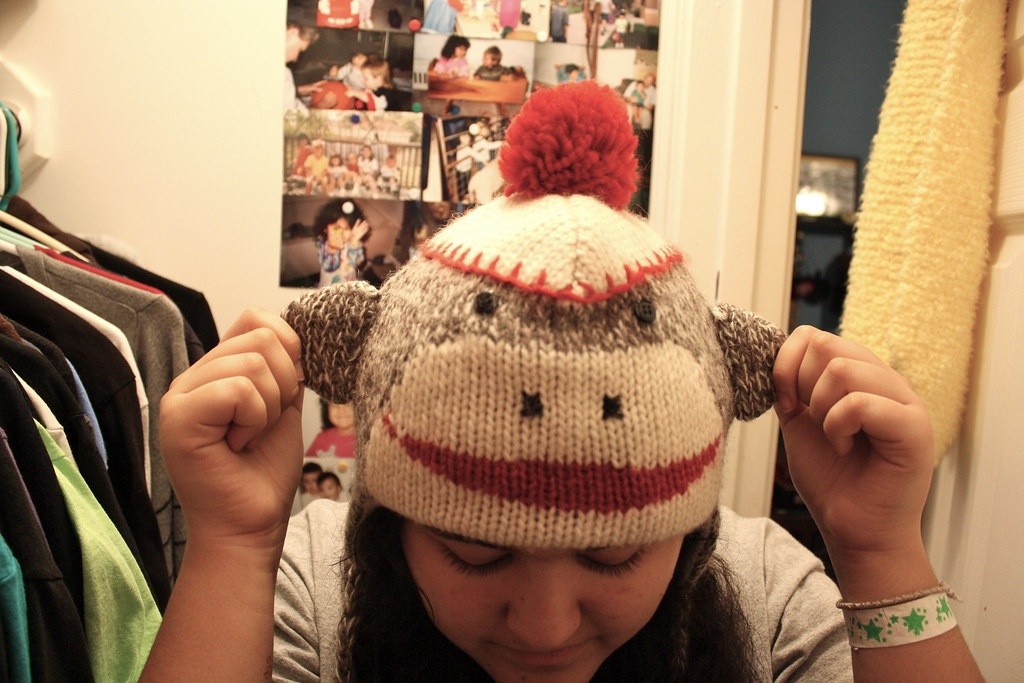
0;100;91;274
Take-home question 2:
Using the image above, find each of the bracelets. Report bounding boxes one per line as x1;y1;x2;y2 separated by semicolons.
835;582;967;609
843;592;957;649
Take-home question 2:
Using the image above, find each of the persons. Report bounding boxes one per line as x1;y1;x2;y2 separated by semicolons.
140;79;986;683
278;0;663;289
299;391;360;512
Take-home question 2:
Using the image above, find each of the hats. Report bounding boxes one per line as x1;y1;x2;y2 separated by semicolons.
279;78;788;550
